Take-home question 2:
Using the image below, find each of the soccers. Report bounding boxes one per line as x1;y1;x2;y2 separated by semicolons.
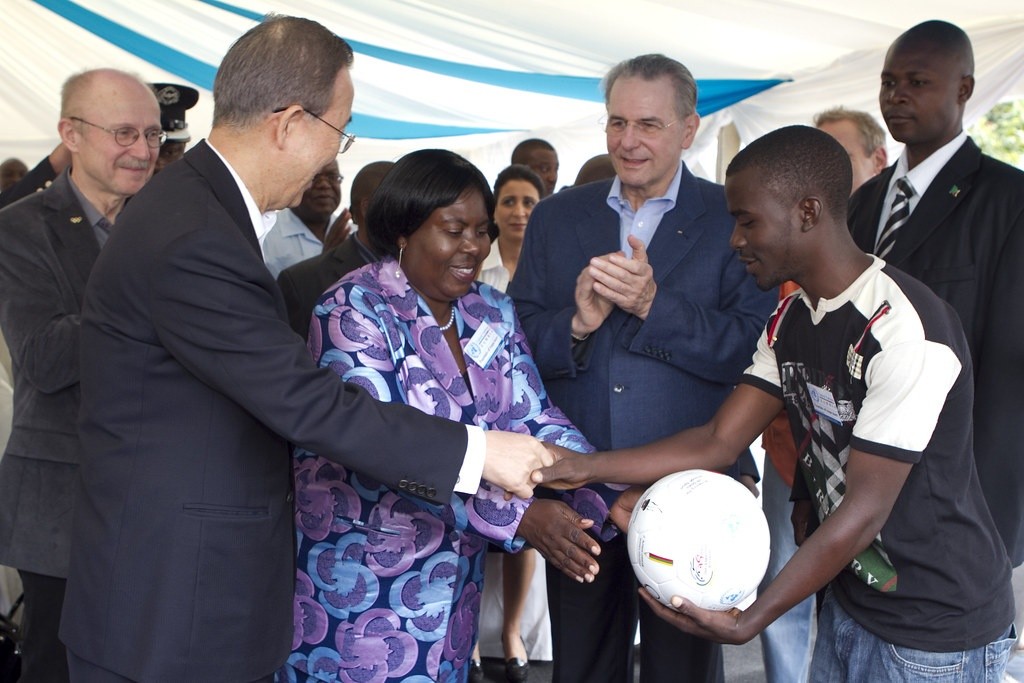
627;468;772;615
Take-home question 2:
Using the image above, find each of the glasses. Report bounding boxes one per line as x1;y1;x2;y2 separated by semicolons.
312;174;344;185
272;107;356;154
597;112;680;137
71;116;168;148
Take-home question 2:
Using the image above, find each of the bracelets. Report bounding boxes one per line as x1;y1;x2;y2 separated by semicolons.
571;332;590;344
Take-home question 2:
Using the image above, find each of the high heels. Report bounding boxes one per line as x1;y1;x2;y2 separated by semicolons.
466;648;484;683
501;630;529;680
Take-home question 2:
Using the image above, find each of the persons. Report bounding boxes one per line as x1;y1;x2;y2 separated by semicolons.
784;20;1023;632
0;56;890;683
56;14;552;683
505;126;1017;683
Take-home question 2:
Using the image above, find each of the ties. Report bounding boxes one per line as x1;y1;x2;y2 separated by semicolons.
876;175;917;263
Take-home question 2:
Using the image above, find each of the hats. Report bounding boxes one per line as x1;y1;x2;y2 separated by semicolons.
146;83;199;143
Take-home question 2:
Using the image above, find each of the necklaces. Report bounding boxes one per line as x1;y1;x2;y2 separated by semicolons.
440;302;455;332
